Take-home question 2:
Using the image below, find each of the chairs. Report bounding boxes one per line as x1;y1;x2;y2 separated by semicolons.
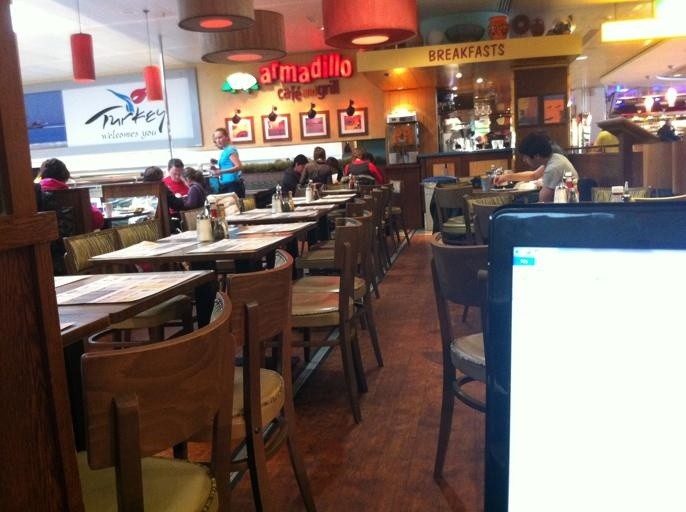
34;173;410;512
431;171;685;479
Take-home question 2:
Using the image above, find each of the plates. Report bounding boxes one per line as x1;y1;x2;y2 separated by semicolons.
384;164;423;229
417;151;511;179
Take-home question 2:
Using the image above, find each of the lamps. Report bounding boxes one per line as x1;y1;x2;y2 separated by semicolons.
176;1;255;34
436;76;516;154
200;11;288;66
346;99;355;116
643;77;656;112
321;0;418;51
70;0;96;82
665;65;678;108
601;0;685;42
308;102;316;119
142;10;162;102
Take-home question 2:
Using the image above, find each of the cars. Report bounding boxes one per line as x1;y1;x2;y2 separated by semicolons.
102;202;112;217
498;140;504;148
480;175;489;192
491;139;498;149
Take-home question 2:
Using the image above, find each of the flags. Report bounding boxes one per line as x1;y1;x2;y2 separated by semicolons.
488;16;508;39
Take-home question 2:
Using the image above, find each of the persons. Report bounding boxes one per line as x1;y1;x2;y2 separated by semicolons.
142;167;190;233
493;132;580;203
162;158;189;214
38;158;105;233
324;156;339;167
344;146;384;185
658;118;683;142
180;166;209;209
282;154;309;194
300;147;340;185
207;128;244;197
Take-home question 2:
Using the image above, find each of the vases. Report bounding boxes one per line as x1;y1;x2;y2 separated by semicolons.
488;16;508;39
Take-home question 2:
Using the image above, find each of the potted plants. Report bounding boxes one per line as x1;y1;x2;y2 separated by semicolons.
225;104;368;145
20;68;203;161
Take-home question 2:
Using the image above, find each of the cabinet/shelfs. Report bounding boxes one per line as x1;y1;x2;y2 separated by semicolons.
271;183;294;213
384;164;423;229
553;172;579;204
196;201;230;242
306;180;319;202
611;180;632;202
417;151;511;179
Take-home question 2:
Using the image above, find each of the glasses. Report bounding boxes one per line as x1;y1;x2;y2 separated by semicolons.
70;0;96;82
346;99;355;116
176;1;255;34
200;11;288;66
142;10;162;102
308;102;316;119
321;0;418;51
601;0;685;42
436;76;516;154
643;77;656;112
665;65;678;108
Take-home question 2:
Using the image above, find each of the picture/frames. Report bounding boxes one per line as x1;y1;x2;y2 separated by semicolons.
225;104;368;145
20;68;203;161
